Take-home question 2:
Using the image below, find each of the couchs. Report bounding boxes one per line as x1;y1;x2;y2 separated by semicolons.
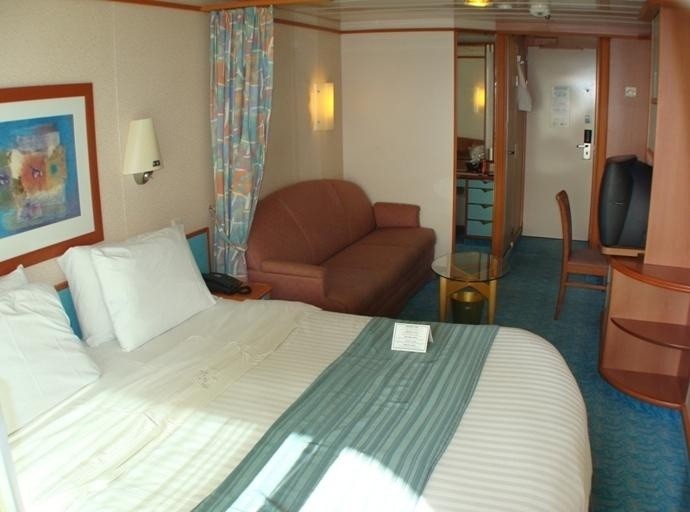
246;180;436;317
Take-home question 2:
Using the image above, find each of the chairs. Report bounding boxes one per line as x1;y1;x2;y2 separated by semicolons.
554;189;610;320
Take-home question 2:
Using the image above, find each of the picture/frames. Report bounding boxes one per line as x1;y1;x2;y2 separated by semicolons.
0;82;104;277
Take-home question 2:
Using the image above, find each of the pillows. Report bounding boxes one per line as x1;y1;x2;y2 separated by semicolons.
0;262;29;297
90;224;216;352
0;281;101;437
56;246;116;348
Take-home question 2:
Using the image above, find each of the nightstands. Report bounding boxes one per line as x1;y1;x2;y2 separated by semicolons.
210;281;274;302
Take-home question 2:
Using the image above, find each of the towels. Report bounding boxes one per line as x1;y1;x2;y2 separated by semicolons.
517;64;532;112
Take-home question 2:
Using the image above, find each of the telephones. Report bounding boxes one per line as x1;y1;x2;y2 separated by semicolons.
201;272;245;295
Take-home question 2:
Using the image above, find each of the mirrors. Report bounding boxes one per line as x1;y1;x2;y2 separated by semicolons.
455;41;496;172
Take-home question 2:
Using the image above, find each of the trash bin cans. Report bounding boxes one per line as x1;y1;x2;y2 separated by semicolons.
451;291;483;326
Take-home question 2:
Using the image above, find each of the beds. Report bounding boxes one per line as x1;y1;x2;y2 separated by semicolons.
0;216;593;509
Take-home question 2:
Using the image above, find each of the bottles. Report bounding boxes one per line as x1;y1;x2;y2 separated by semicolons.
481;159;489;175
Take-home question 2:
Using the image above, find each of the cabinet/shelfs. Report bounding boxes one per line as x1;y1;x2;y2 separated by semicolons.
465;179;494;239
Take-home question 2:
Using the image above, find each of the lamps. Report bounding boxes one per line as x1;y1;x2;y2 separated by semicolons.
123;117;164;185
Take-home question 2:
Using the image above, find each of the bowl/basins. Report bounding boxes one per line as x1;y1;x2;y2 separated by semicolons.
465;160;479;174
